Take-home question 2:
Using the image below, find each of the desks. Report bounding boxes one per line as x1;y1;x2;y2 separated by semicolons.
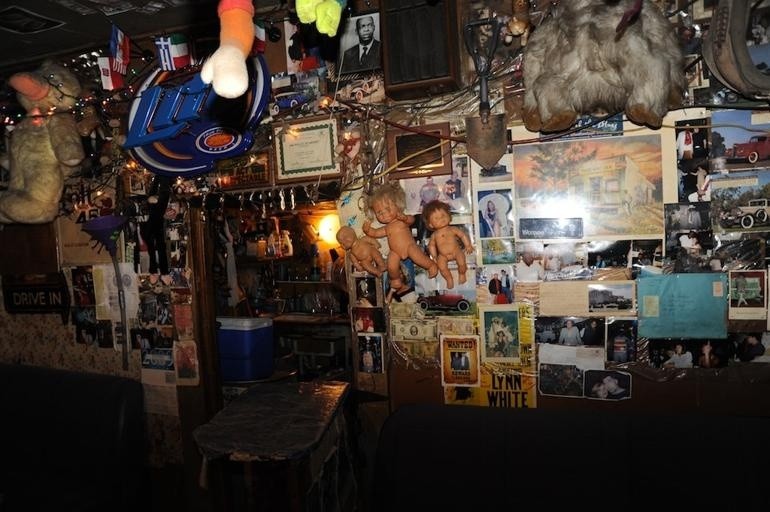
189;379;353;510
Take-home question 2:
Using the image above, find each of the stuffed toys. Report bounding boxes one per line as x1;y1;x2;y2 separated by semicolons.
521;0;687;136
201;1;258;99
504;0;531;47
1;60;86;225
296;0;351;37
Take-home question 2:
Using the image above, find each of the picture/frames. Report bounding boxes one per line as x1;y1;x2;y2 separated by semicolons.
273;111;345;186
385;122;453;180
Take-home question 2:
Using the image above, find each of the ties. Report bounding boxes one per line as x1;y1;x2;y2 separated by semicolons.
360;47;368;64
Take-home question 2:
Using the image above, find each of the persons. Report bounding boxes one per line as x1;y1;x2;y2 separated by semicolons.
363;184;438;288
420;172;465;209
423;199;474;289
338;226;387;277
341;16;380;72
483;123;770;400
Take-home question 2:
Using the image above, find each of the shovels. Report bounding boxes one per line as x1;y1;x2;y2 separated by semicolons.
462;17;508;171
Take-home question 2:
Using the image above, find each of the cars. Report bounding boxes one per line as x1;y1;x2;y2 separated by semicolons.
723;134;770;163
718;198;770;229
